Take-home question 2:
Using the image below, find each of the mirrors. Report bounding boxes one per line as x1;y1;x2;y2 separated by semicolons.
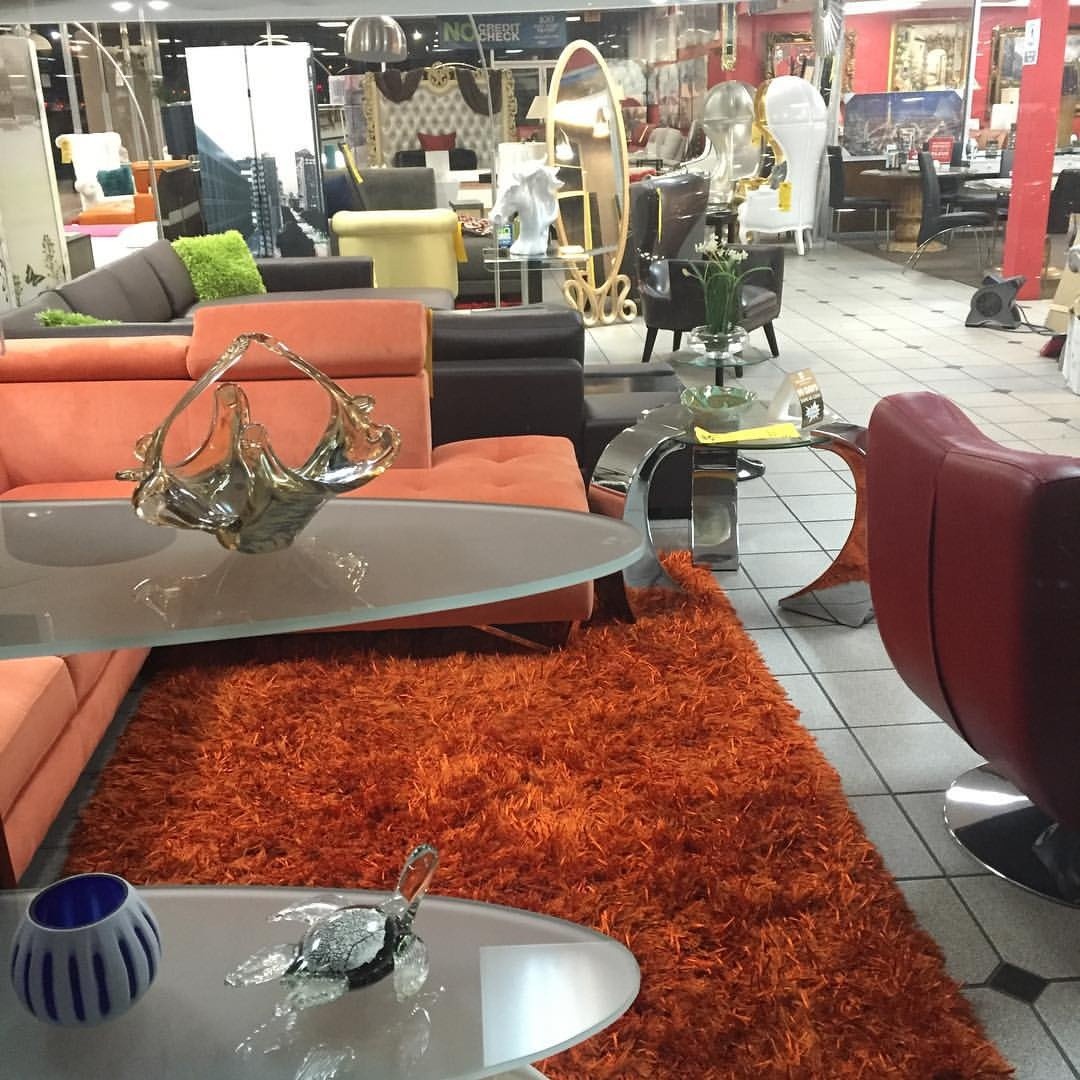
546;39;637;327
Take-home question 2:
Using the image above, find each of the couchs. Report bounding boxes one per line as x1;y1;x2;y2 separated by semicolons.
0;237;593;887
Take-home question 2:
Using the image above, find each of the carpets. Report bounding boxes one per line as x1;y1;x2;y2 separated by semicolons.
61;550;1015;1080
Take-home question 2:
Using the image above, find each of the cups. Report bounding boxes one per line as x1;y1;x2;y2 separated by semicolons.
7;872;159;1028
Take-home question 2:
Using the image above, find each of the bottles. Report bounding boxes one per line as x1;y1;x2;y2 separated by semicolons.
985;141;997;159
1008;122;1016;150
887;143;899;169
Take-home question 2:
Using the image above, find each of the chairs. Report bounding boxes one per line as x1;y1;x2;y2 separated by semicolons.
56;131;190;225
868;390;1080;912
628;76;1080;378
322;148;476;297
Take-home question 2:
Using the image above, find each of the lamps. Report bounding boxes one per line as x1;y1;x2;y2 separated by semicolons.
345;14;500;308
27;30;52;50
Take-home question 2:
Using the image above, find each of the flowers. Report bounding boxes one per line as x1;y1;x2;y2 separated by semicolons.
683;233;773;334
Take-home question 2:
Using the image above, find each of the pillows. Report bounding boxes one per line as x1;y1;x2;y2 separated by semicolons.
97;167;134;196
33;309;124;327
171;229;267;303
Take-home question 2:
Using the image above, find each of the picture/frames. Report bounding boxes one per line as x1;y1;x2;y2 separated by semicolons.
720;2;1080;119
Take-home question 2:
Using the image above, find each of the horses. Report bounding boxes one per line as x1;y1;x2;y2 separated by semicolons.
488;151;565;256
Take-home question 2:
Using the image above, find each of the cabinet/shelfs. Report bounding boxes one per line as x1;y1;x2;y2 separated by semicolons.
0;498;644;1080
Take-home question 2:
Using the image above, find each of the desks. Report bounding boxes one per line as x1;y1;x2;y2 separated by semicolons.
963;180;1011;266
858;170;962;255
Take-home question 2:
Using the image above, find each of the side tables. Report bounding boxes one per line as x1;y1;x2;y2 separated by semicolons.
587;404;875;628
